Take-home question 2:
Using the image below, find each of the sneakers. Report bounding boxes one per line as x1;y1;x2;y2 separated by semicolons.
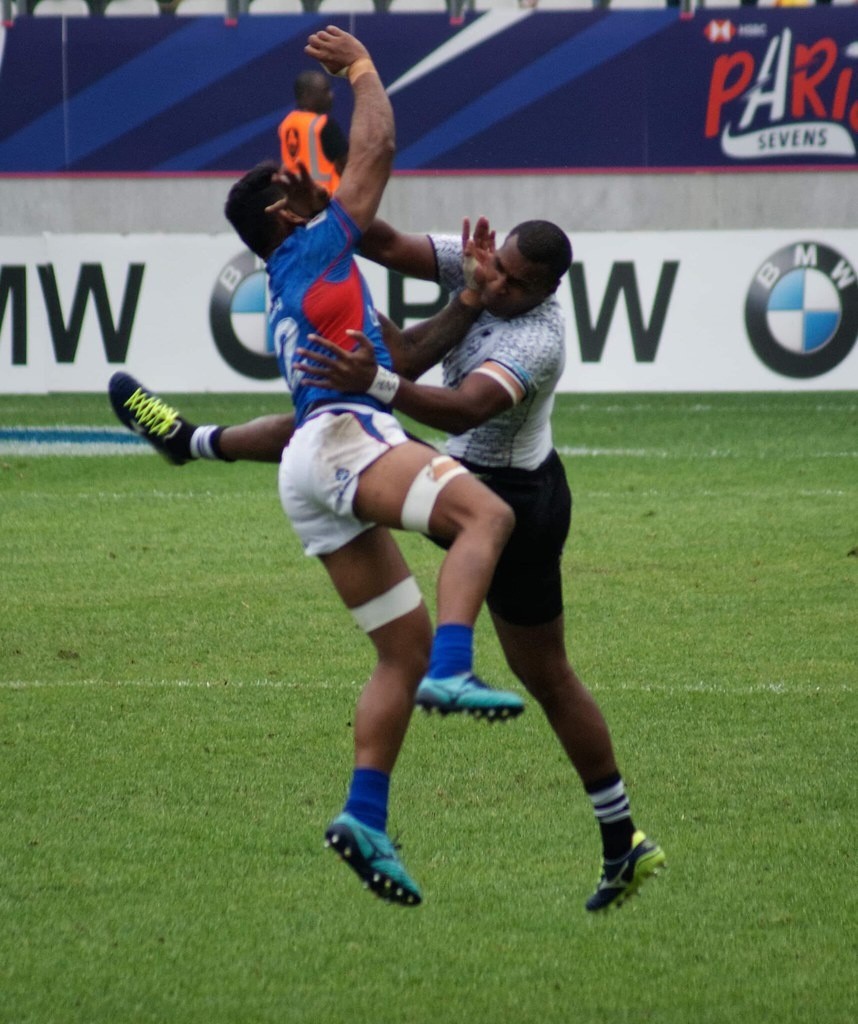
585;829;668;916
325;811;424;907
110;371;197;467
416;671;527;724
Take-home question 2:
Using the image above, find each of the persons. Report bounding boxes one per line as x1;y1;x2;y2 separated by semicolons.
107;159;668;914
224;22;526;907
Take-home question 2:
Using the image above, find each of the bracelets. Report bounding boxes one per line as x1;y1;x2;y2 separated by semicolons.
366;365;400;404
347;57;377;86
459;287;485;309
